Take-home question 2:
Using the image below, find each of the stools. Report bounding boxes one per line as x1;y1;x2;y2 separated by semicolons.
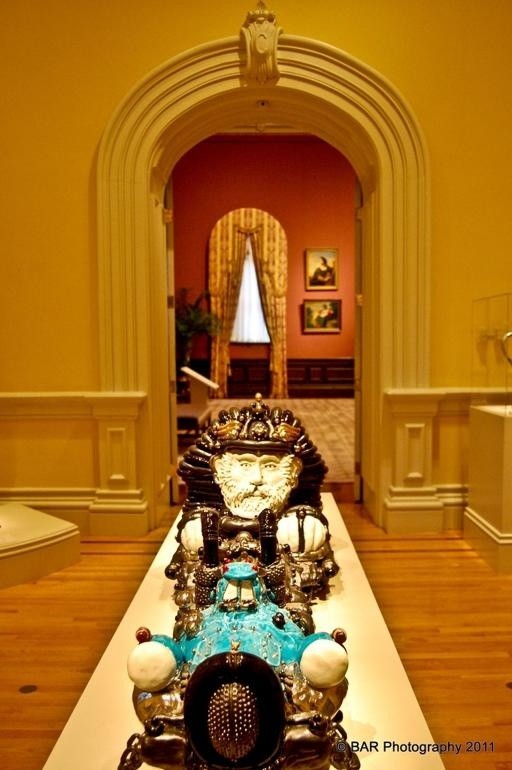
177;406;213;444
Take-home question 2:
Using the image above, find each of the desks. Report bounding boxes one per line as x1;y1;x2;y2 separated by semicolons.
42;485;452;770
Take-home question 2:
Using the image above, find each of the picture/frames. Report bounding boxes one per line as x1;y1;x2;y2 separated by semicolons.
302;298;343;335
304;247;340;291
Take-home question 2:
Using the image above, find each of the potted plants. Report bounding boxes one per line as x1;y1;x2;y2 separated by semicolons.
175;287;226;404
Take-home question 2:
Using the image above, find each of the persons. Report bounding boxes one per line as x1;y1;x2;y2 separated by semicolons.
208;443;303;520
309;255;335;286
314;303;335;327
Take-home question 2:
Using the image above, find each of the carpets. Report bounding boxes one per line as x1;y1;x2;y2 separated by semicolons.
206;396;356;487
